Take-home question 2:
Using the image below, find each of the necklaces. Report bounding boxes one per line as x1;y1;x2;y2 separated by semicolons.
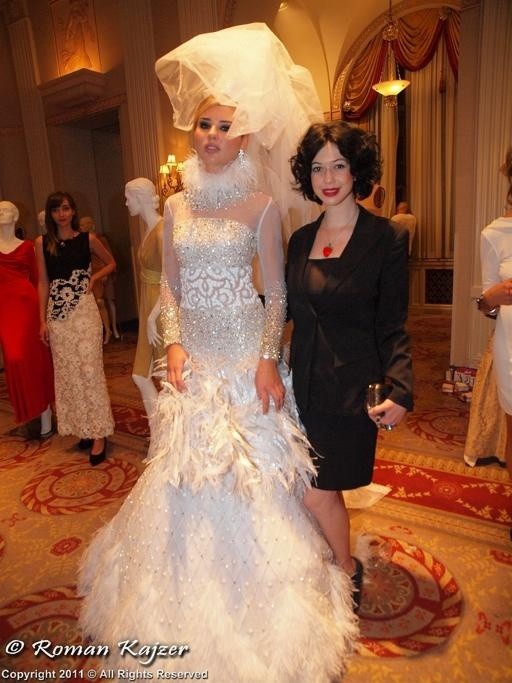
323;204;358;257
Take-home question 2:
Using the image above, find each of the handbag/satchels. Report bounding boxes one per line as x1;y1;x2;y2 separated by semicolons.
364;384;391;430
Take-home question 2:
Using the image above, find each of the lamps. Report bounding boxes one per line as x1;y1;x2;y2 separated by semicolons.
159;153;183;199
371;1;410;109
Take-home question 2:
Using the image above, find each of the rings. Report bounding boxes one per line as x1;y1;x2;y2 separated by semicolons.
387;423;392;431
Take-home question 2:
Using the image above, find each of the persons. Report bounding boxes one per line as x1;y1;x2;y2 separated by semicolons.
389;201;417;257
461;141;512;483
0;200;55;442
36;210;47;236
72;21;367;683
123;178;165;463
98;216;121;346
79;216;113;345
33;192;117;468
255;121;415;612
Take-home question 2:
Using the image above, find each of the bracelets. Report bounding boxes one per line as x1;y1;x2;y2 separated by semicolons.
478;291;500;317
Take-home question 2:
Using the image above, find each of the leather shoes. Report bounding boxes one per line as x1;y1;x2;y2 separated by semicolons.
350;556;363;612
89;438;107;465
78;437;92;448
